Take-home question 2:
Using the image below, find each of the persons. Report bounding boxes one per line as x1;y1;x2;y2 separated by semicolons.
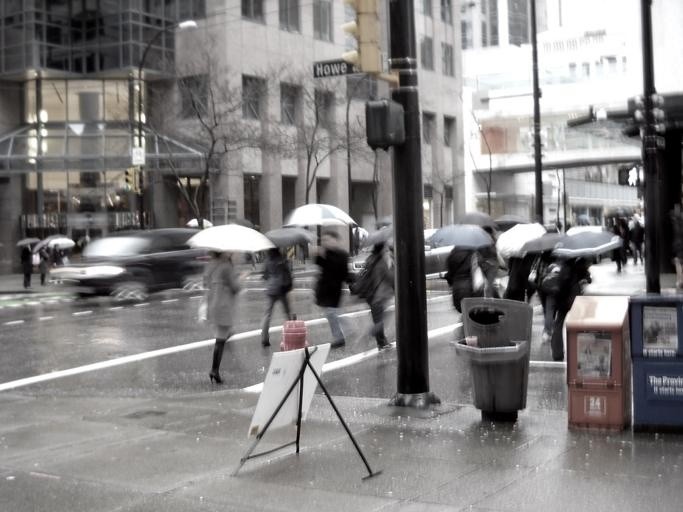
17;248;35;288
355;243;396;349
310;232;352;351
259;248;296;347
442;226;591;362
610;213;645;270
54;244;66;285
198;251;249;388
39;249;48;284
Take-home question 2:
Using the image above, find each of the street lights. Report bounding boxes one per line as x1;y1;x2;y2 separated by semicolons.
135;19;200;230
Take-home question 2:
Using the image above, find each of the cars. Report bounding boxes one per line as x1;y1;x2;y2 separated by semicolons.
343;227;455;285
46;226;217;306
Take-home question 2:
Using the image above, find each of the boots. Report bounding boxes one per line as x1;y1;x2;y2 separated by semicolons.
209;338;225;385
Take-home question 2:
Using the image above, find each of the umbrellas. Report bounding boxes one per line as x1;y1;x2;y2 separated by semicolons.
428;211;623;258
186;205;393;256
16;233;76;252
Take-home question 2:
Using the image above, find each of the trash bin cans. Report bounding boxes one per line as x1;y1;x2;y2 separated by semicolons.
454;297;532;423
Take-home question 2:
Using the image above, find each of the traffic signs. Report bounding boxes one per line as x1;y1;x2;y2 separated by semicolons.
313;60;359;77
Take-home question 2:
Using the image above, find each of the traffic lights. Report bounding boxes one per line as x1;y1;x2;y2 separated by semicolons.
337;1;386;75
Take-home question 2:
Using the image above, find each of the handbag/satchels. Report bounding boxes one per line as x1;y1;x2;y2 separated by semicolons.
470;251;487;294
527;269;543;290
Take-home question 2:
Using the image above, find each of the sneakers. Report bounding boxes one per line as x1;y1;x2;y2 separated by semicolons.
377;343;392;351
331;341;345;347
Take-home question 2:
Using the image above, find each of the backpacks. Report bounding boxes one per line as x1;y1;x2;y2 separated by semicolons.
539;263;562;295
350;256;388;300
